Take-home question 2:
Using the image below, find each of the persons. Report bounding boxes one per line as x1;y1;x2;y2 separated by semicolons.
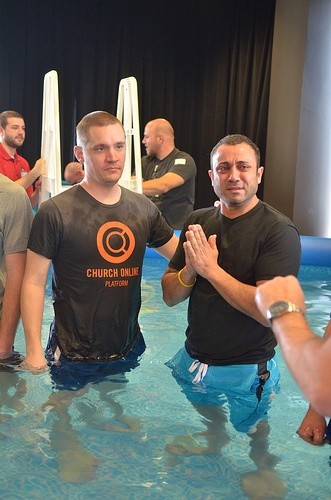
0;173;34;361
0;110;47;207
255;275;331;447
132;119;199;231
65;161;85;185
160;135;301;392
19;111;180;375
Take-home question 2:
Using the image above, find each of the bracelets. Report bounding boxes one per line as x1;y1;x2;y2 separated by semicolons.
177;268;196;288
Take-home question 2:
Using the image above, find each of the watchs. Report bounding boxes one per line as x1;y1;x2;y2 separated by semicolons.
266;301;305;326
